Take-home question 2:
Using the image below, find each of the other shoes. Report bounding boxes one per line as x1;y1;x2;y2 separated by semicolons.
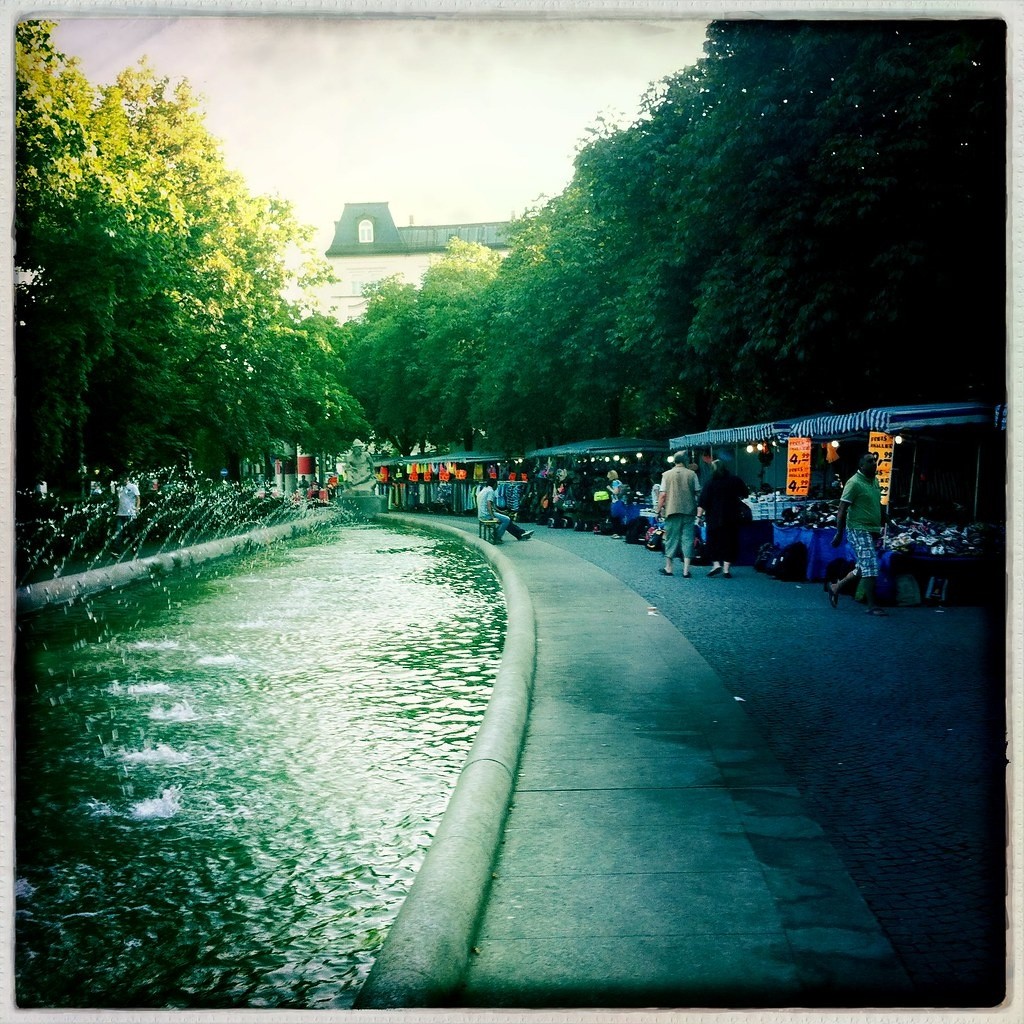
876;517;984;556
743;490;809;502
706;567;722;577
610;534;623;539
520;530;534;538
491;532;503;544
723;573;732;579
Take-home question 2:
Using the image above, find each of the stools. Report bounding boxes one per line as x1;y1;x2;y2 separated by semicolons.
479;521;501;542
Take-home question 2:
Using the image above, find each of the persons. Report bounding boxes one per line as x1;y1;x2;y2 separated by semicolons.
649;473;661;510
655;450;702;578
607;470;622;539
345;438;377;486
477;478;535;543
825;451;890;617
310;477;319;486
696;458;750;579
298;475;309;488
110;472;140;559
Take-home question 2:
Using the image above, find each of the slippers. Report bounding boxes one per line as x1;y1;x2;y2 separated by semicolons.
682;572;691;578
866;608;888;616
659;568;673;576
827;582;838;608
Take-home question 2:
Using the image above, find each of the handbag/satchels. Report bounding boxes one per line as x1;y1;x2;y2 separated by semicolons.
736;497;752;528
610;495;630;518
547;516;572;528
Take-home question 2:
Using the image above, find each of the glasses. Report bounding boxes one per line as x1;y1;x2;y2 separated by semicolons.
861;458;876;466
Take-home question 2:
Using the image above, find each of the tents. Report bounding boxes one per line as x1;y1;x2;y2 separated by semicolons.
790;400;986;524
669;413;834;519
372;449;523;516
526;436;666;475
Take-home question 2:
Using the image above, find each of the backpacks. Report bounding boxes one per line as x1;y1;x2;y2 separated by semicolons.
626;516;666;550
754;541;808;582
677;537;712;565
823;558;892;605
615;480;631;500
